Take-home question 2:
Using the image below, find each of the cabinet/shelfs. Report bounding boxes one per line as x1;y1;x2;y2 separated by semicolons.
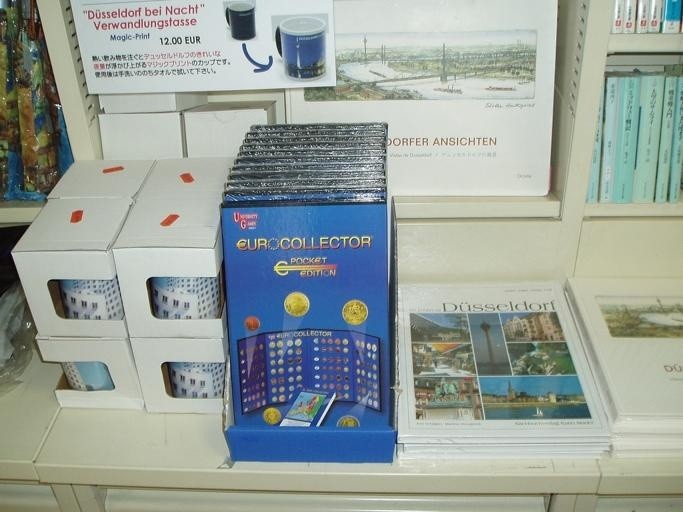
33;483;578;512
43;1;615;287
1;480;82;512
575;494;682;512
554;0;683;278
1;0;99;224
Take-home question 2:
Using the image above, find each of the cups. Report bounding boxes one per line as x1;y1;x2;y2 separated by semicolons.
276;17;327;81
226;2;256;39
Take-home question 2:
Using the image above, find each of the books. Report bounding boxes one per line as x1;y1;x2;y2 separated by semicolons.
610;0;682;33
584;51;682;204
393;275;683;462
